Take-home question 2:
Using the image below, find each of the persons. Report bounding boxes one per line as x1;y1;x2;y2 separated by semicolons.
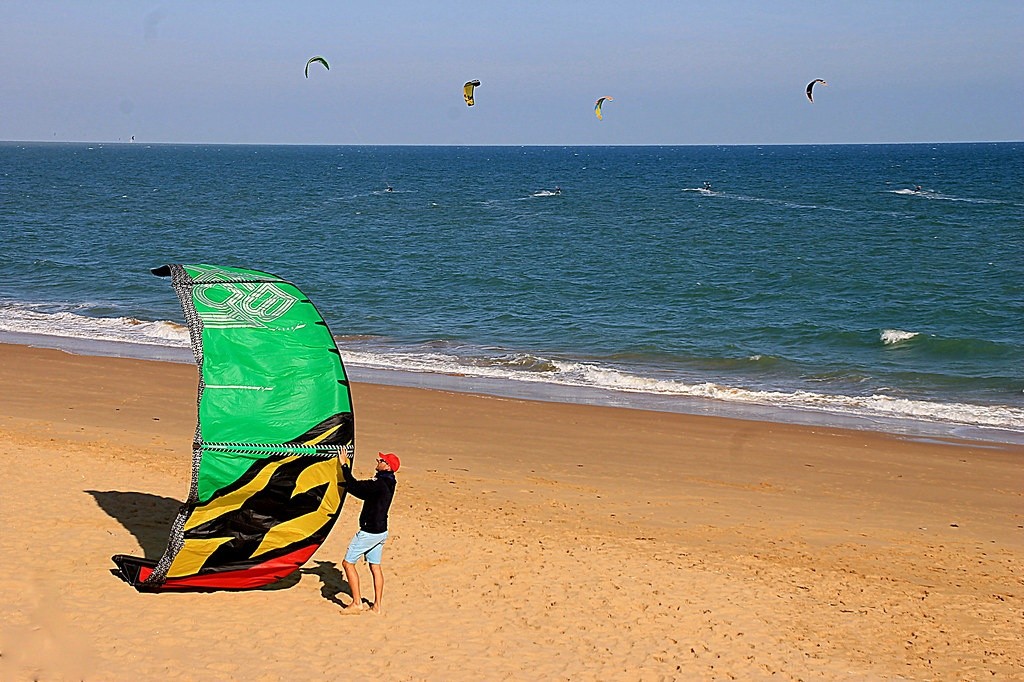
336;445;400;615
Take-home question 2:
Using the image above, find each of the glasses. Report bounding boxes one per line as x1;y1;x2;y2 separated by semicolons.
376;459;385;462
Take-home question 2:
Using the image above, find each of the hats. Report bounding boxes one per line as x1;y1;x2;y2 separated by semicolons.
379;452;401;472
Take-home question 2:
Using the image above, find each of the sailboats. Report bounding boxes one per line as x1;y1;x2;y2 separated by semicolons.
110;260;357;592
